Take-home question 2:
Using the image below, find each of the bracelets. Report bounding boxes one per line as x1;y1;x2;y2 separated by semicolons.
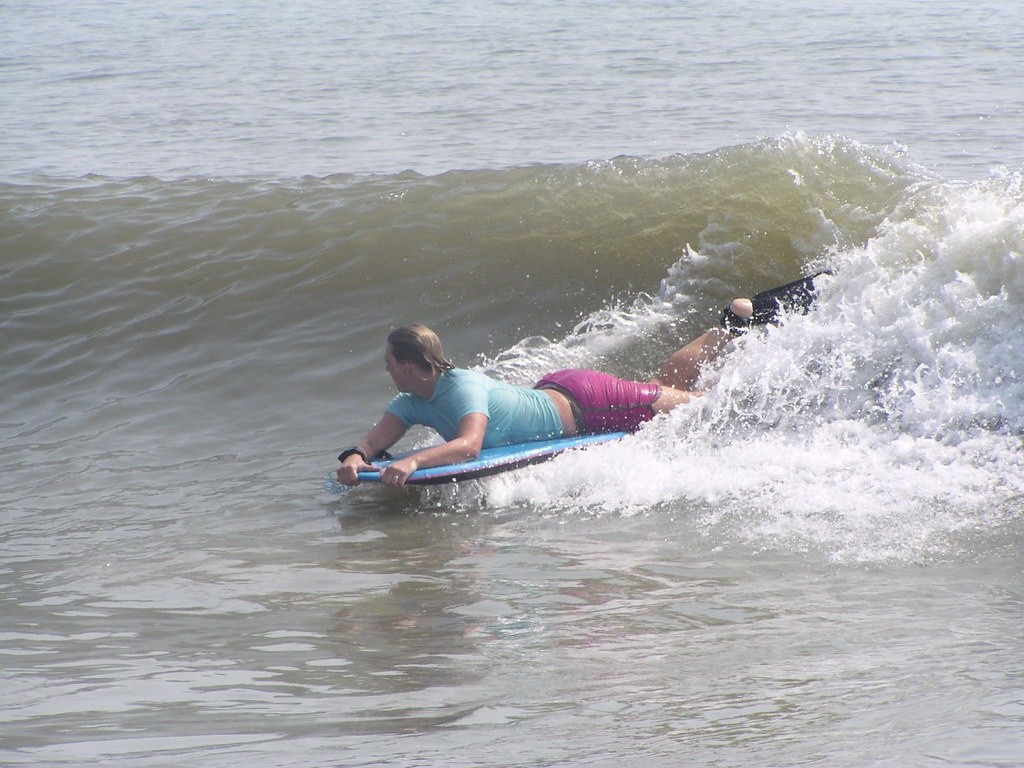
338;446;366;465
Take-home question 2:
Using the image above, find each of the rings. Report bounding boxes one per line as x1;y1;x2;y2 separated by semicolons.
393;476;399;478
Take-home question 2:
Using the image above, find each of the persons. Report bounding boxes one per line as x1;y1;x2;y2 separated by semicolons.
337;274;830;492
